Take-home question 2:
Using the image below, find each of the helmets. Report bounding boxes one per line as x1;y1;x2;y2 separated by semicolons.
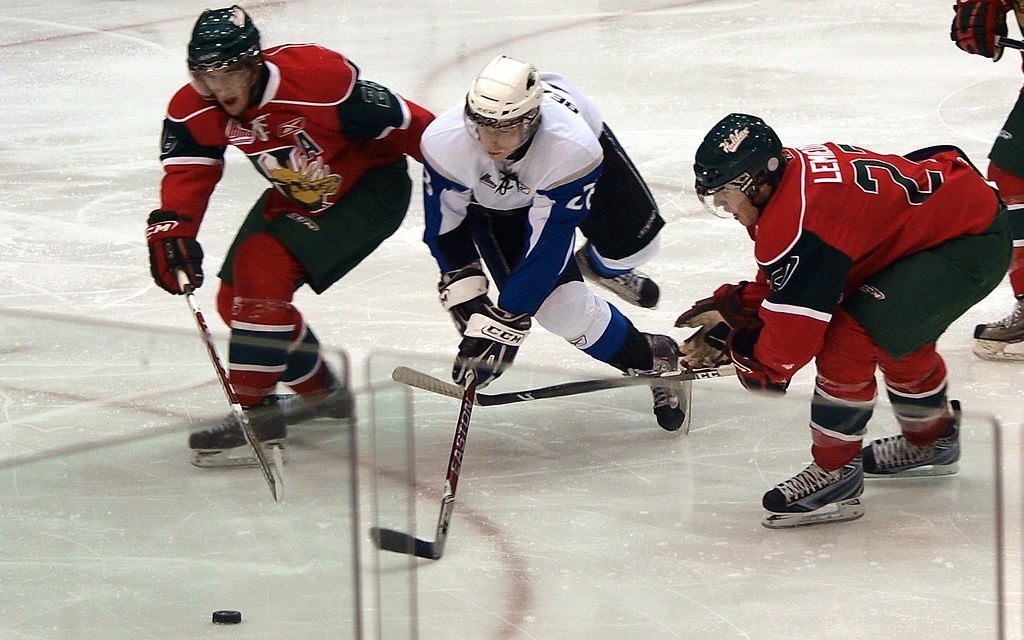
186;4;261;72
464;52;543;130
694;113;782;197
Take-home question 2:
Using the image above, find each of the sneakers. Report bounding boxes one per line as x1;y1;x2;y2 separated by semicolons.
974;293;1024;361
643;333;691;434
862;400;961;478
573;239;659;308
761;455;865;529
272;384;354;424
189;402;287;469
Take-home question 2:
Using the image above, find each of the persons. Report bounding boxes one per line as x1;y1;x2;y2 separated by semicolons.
419;56;693;437
677;113;1012;529
951;0;1024;364
145;5;436;470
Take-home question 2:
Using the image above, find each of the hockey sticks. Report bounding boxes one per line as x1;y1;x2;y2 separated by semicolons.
392;363;739;408
173;265;287;504
367;366;476;560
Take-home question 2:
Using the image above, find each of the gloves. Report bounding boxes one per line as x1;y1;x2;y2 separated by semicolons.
950;0;1014;62
451;303;532;392
726;331;791;396
437;260;494;338
145;209;204;295
674;280;749;333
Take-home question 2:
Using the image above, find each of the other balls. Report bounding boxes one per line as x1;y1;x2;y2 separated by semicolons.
211;609;242;625
